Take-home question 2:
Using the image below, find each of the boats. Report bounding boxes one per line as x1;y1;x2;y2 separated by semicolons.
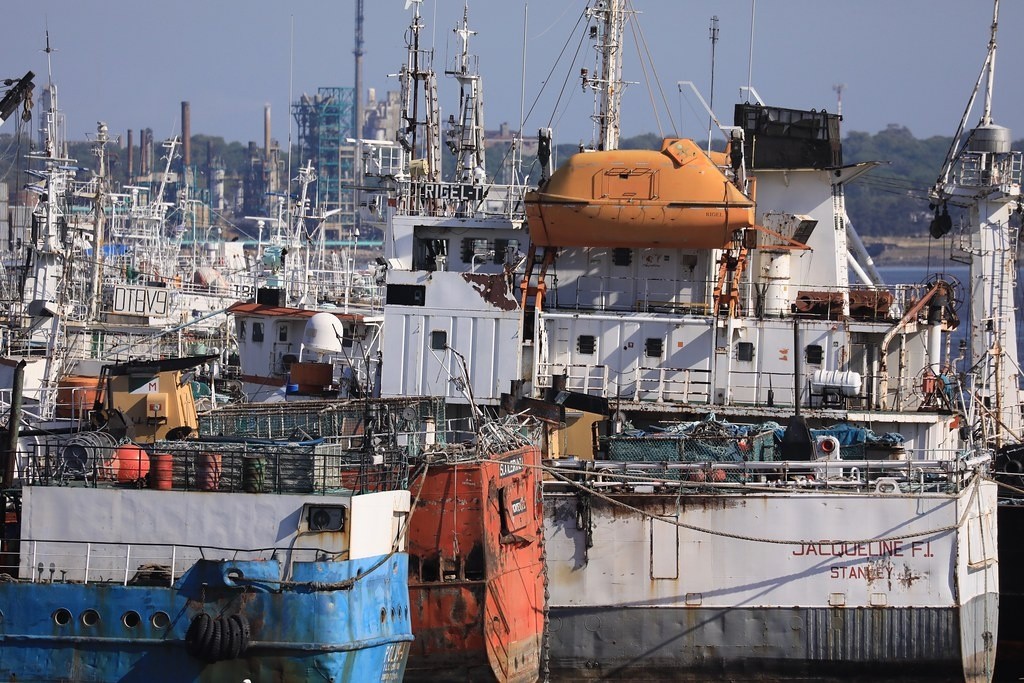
0;4;1023;683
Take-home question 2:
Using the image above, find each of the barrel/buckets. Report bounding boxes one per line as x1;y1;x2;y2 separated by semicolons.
243;455;265;492
149;454;172;490
197;452;221;490
